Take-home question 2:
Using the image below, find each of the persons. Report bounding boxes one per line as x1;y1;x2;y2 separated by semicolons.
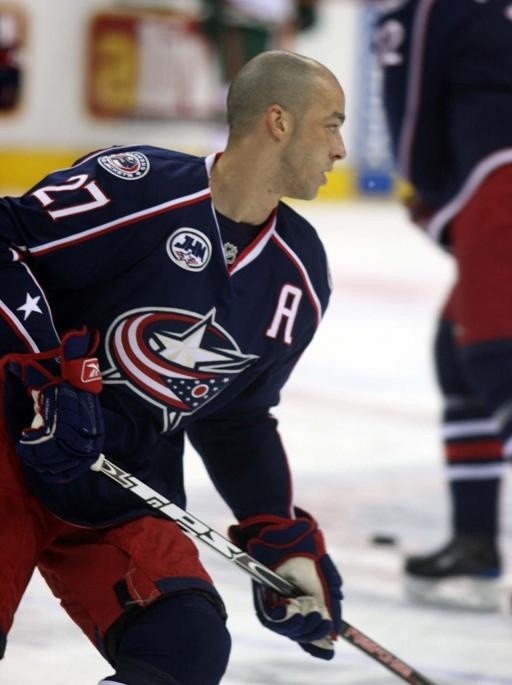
0;41;353;685
341;0;511;589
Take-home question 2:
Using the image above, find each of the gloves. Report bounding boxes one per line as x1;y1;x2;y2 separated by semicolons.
1;324;106;504
229;504;343;659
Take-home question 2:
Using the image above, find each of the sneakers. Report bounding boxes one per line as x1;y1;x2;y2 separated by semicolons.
406;531;501;577
